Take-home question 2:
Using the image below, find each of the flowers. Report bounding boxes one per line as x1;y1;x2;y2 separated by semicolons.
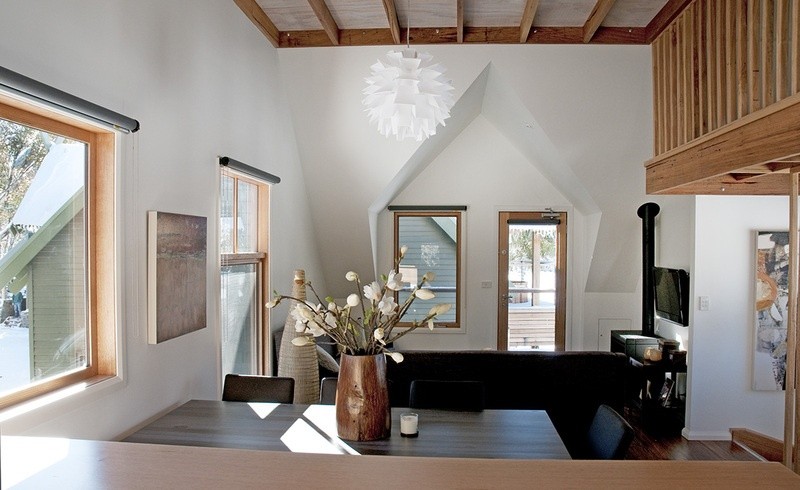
265;245;451;364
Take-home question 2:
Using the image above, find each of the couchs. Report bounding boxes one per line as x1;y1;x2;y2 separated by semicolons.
388;349;627;429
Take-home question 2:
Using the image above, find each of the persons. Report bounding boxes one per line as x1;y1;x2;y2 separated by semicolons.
12;289;23;318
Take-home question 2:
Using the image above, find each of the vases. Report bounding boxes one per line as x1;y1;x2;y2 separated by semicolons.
335;348;392;442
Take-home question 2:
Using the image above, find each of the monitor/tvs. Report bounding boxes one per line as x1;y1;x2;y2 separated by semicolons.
651;267;689;327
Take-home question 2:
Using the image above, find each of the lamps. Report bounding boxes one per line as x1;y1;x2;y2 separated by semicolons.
362;0;454;141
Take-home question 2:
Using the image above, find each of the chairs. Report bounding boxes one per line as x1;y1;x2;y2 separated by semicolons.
221;374;295;404
575;404;633;460
409;379;484;410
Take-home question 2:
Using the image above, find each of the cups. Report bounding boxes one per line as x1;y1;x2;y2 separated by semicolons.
399;412;418;434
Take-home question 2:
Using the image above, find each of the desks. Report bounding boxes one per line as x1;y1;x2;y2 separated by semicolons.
121;399;573;459
